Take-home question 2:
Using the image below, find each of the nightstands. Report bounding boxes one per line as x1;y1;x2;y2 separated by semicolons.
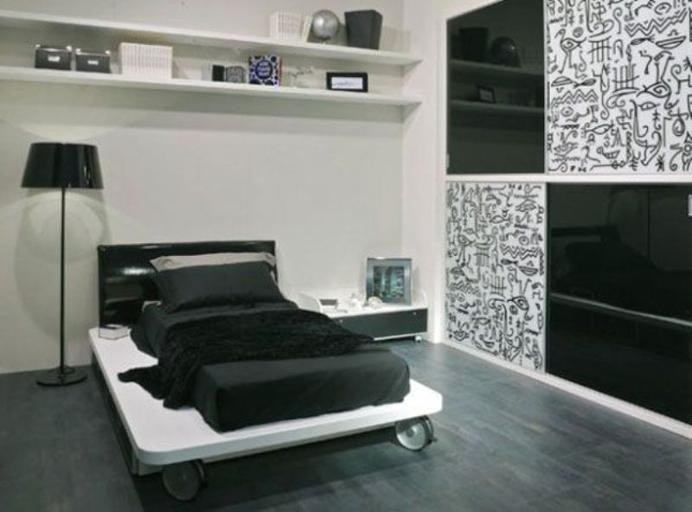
300;294;429;345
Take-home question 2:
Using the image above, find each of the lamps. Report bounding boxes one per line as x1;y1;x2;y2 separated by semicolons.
19;141;105;388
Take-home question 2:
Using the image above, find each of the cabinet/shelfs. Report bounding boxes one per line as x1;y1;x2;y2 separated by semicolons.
448;57;543;115
0;8;424;108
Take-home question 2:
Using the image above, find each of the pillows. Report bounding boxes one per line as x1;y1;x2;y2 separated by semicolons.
147;250;290;314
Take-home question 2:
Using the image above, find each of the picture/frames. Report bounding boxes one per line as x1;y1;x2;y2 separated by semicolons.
365;257;413;306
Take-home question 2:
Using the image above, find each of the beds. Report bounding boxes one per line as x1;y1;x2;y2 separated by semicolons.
86;238;447;502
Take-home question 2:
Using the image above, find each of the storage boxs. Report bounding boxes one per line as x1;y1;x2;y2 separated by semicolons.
33;44;112;73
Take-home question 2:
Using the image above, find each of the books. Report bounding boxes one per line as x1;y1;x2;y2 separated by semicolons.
118;41;173;79
270;11;313;42
